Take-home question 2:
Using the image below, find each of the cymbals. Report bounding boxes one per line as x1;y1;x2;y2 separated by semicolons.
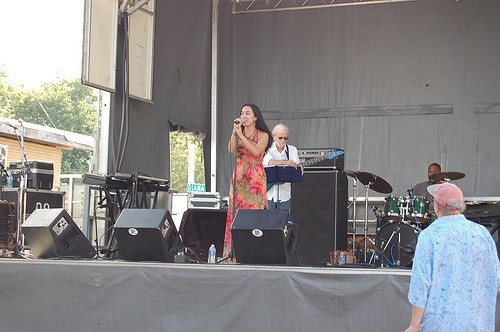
356;170;392;195
343;168;358;178
427;172;466;183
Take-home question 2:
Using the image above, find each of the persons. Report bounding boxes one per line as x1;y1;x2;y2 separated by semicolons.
262;123;304;210
223;103;273;264
405;183;500;332
413;163;443;230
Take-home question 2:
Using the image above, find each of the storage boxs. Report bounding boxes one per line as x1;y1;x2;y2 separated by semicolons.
8;161;53;190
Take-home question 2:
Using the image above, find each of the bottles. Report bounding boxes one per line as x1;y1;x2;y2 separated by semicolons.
208;245;216;263
6;172;13;188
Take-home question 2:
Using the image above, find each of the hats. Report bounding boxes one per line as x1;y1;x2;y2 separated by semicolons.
427;182;465;210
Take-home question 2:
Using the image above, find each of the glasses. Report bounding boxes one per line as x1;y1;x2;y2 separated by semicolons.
274;134;289;140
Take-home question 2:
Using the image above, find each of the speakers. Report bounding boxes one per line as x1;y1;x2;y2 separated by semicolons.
230;208;296;265
289;169;348;267
21;208;99;260
113;209;186;263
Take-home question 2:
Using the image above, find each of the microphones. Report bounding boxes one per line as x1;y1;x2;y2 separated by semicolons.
234;121;240;124
405;248;412;253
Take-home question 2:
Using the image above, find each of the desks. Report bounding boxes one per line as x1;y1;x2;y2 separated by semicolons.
178;208;227;263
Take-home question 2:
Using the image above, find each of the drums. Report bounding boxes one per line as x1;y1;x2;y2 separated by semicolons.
373;220;420;267
381;195;405;221
407;195;431;225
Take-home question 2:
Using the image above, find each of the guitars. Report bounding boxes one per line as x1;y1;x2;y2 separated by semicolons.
264;149;345;189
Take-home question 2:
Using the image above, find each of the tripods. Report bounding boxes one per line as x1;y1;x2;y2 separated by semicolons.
346;180;376;262
5;120;32;261
